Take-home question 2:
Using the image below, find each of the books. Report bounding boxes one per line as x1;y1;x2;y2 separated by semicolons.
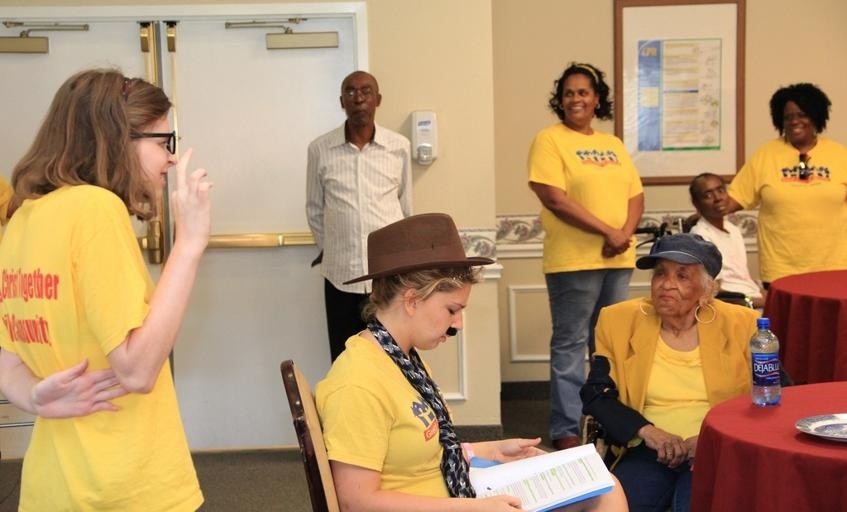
478;444;614;512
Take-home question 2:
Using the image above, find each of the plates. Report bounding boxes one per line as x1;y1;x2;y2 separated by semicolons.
794;413;847;442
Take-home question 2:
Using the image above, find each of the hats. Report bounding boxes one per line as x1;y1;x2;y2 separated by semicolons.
342;213;495;286
636;232;722;280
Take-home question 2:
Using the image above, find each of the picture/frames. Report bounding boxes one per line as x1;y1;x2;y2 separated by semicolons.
613;0;746;184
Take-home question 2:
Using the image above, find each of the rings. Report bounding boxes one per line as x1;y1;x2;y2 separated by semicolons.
667;452;675;456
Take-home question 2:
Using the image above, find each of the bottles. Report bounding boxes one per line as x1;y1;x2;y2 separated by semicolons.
749;318;782;408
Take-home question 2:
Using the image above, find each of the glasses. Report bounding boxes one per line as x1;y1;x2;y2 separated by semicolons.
129;130;176;155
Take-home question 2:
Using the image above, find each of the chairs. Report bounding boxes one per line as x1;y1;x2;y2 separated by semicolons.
580;361;793;476
280;358;338;511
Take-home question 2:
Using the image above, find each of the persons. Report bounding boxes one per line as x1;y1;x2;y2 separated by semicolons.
687;84;847;289
579;233;762;512
1;68;216;512
527;60;645;452
315;212;632;512
306;71;413;362
687;174;767;311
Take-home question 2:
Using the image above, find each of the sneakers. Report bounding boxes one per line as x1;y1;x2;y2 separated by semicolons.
553;435;581;450
